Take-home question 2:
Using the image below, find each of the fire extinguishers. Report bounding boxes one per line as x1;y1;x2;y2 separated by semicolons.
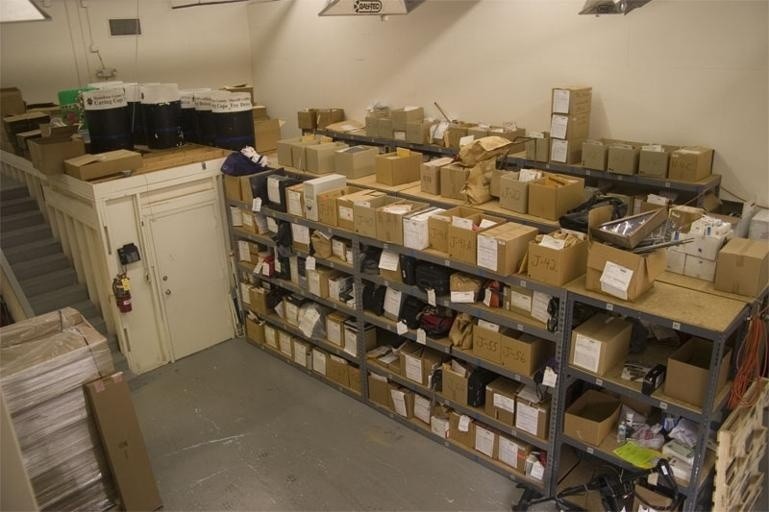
111;273;132;313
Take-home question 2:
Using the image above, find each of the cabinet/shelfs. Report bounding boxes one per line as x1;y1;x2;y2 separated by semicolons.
226;197;569;502
400;187;561;242
657;268;768;328
521;157;723;214
264;153;429;196
551;276;752;512
302;126;520;177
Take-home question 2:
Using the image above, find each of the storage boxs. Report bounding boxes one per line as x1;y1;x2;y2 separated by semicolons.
251;104;269;121
364;105;390;136
316;185;364;227
276;136;302;167
259;279;274;291
401;205;448;253
245;317;264;344
563;389;625;450
327;273;353;299
638;142;680;181
5;111;29;143
288;253;299;287
448;272;481;305
0;305;84;348
633;194;647;216
326;356;350;387
85;370;163;512
42;478;117;511
263;323;279;349
567;312;635;377
514;385;551;441
280;295;299;327
585;239;662;305
531;289;551;325
293;339;312;368
379;248;403;286
265;173;298;212
419;157;454;196
499;328;546;377
311;230;333;258
234;240;261;254
254;117;282;153
527;172;587;220
265;215;290;244
467;122;489;140
580;137;615;172
534;131;549;163
473;421;500;460
695;193;723;215
324;311;350;348
277;329;295;358
499;171;527;212
297;254;308;290
383;284;404;323
223;174;243;202
274;248;290;279
297;106;316;128
261;255;276;276
229;206;243;228
399;253;415;284
298;301;335;341
489;125;526;154
606;137;650;176
445;213;508;268
301;173;347;222
248;285;280;316
444;126;468;148
21;421;97;481
241;168;272;203
26;133;84;173
550;139;586;164
414;260;453;298
390;107;423;142
551;86;594;115
497;433;534;477
368;375;397;409
475;221;539;279
336;189;387;234
239;282;252;305
315;108;344;128
665;248;685;276
664;335;735;411
667;144;715;185
427;205;484;255
330;237;362;262
347;364;361;393
22;111;50;129
375;151;423;186
1;323;116;418
290;222;311;252
428;119;464;147
449;409;476;452
306;265;338;299
526;131;536;160
240;209;269;235
640;201;668;214
312;348;327;375
412;390;436;425
352;196;404;243
284;182;306;218
379;114;391;137
342;318;377;357
470;322;509;369
489;170;509;198
402;294;422;327
509;282;534;317
0;86;28;117
387;381;415;420
62;148;143;180
605;185;633;217
551;113;591;140
220;83;255;103
468;368;495;408
397;342;449;390
441;360;474;408
483;376;522;430
714;236;768;300
363;282;385;318
440;162;469;201
529;229;589;288
375;197;431;246
11;386;94;457
14;128;41;149
235;252;259;263
431;415;450;439
406;119;433;145
333;144;379;179
39;121;80;143
684;233;717;261
683;253;717;281
631;482;674;512
291;135;334;169
620;393;649;416
31;449;104;510
306;351;313;369
304;142;349;176
